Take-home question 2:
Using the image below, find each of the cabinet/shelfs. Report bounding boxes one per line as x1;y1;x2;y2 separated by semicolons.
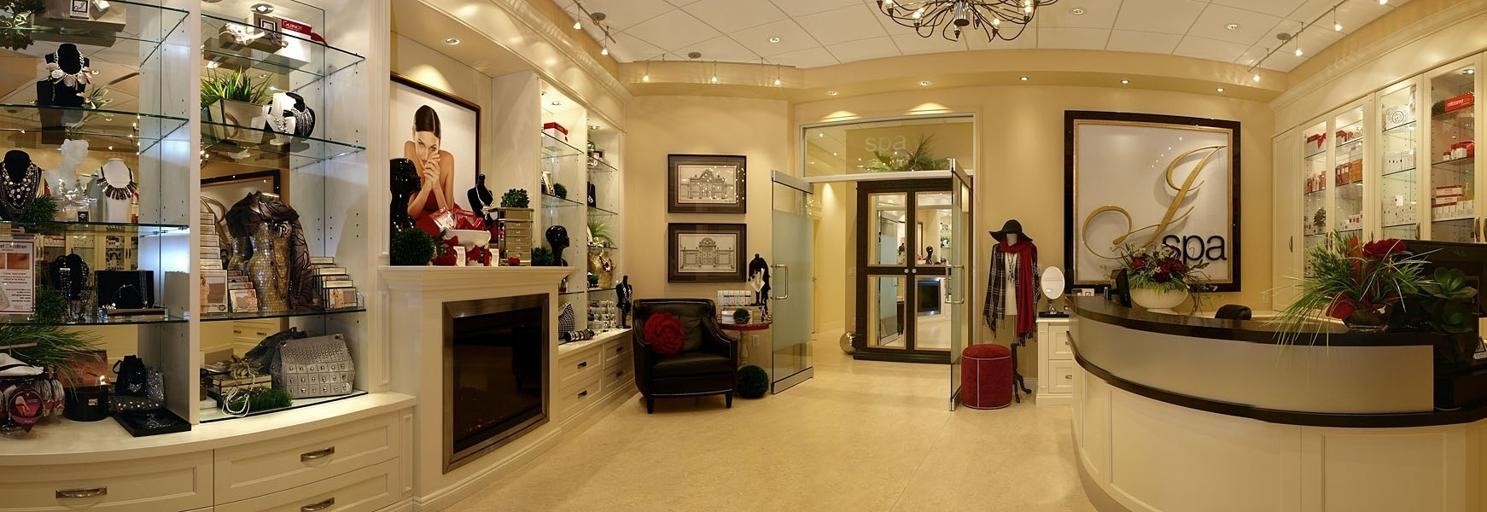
0;2;374;423
1034;319;1073;401
1271;51;1486;321
0;391;414;511
490;71;635;421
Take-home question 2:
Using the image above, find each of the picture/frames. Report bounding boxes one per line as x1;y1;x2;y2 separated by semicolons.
667;153;747;284
389;72;482;237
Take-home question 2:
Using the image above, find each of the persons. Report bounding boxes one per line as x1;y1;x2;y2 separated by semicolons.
390;157;421;231
746;252;770;305
545;225;569;266
921;245;934;264
981;219;1040;346
0;353;44;377
402;105;462;237
896;241;905;335
614;273;634;330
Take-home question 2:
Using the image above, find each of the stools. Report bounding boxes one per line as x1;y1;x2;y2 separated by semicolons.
960;343;1014;410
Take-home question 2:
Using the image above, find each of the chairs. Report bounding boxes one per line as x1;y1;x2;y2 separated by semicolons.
630;298;737;415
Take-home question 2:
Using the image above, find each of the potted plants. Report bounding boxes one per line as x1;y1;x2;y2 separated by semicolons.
1414;266;1476;367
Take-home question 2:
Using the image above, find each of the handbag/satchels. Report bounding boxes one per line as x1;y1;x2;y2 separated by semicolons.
268;333;356;399
53;350;166;421
245;327;307;375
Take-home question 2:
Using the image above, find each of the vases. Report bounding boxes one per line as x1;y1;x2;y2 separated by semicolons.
1128;286;1187;315
1340;309;1385;334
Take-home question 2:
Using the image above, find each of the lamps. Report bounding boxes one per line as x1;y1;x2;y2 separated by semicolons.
875;0;1058;46
570;0;617;57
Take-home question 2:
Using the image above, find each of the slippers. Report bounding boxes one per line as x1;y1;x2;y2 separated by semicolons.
0;361;48;383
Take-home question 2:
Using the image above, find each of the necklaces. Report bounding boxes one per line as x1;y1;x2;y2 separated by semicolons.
271;114;289;140
95;163;138;201
1006;252;1015;283
547;177;554;195
599;255;613;272
620;282;633;304
54;165;89;209
45;46;94;90
56;259;94;323
0;161;40;217
289;102;313;135
391;213;414;233
586;184;594;203
475;184;495;220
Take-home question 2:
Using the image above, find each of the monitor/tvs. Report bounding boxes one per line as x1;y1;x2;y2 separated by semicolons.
1104;266;1133;305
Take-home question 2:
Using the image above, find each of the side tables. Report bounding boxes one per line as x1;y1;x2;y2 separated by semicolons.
716;314;771;367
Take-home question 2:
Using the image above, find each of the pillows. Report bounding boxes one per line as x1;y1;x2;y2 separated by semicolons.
642;312;685;356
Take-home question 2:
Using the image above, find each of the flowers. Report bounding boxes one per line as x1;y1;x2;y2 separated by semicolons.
1112;238;1223;318
1252;227;1443;368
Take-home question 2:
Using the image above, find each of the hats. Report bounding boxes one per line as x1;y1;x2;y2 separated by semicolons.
988;219;1032;243
